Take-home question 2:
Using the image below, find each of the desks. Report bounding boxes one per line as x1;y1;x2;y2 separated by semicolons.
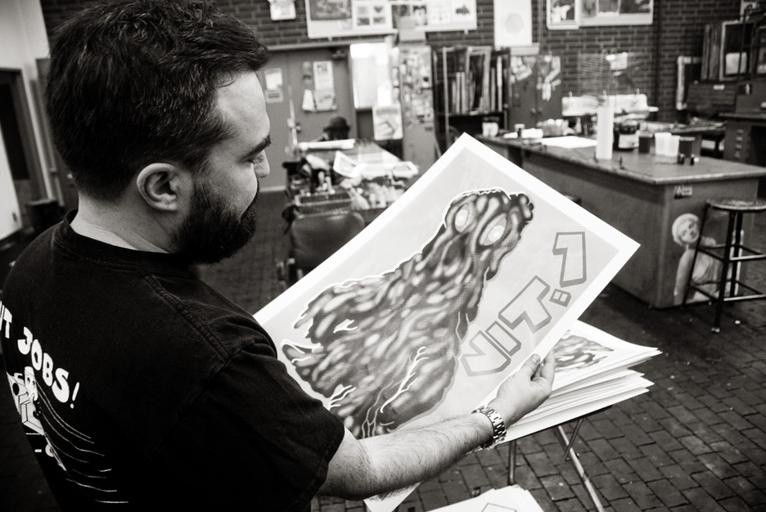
483;129;766;307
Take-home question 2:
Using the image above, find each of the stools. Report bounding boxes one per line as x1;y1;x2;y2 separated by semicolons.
686;195;766;332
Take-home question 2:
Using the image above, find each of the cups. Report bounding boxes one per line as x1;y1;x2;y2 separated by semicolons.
653;132;682;158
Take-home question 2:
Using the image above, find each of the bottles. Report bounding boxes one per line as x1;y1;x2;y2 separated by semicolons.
594;95;618;161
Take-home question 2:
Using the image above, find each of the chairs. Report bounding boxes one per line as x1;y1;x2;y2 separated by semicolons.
287;209;371;282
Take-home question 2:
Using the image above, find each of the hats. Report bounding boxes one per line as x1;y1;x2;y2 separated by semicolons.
323;116;350;131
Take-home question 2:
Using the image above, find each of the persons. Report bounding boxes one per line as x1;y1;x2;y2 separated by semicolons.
0;0;556;510
672;213;721;306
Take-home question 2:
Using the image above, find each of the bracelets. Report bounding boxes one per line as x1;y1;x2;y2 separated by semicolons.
471;406;505;451
